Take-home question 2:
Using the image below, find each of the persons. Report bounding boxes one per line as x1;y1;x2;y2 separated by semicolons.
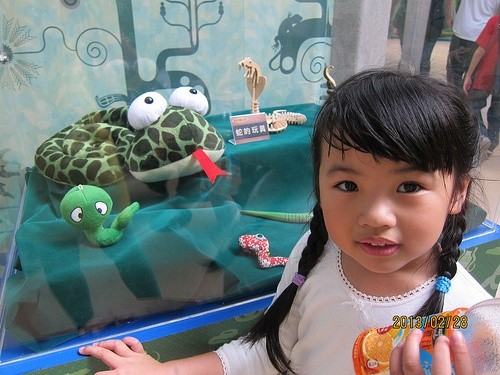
391;0;454;74
462;15;500;164
446;0;500;96
78;67;495;375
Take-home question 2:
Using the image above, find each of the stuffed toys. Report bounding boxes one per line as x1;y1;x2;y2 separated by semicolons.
34;86;232;202
60;184;141;248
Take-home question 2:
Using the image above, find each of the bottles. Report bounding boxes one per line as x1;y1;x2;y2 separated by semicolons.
350;296;500;375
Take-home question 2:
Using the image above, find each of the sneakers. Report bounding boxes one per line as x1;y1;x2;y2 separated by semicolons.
472;132;491;167
